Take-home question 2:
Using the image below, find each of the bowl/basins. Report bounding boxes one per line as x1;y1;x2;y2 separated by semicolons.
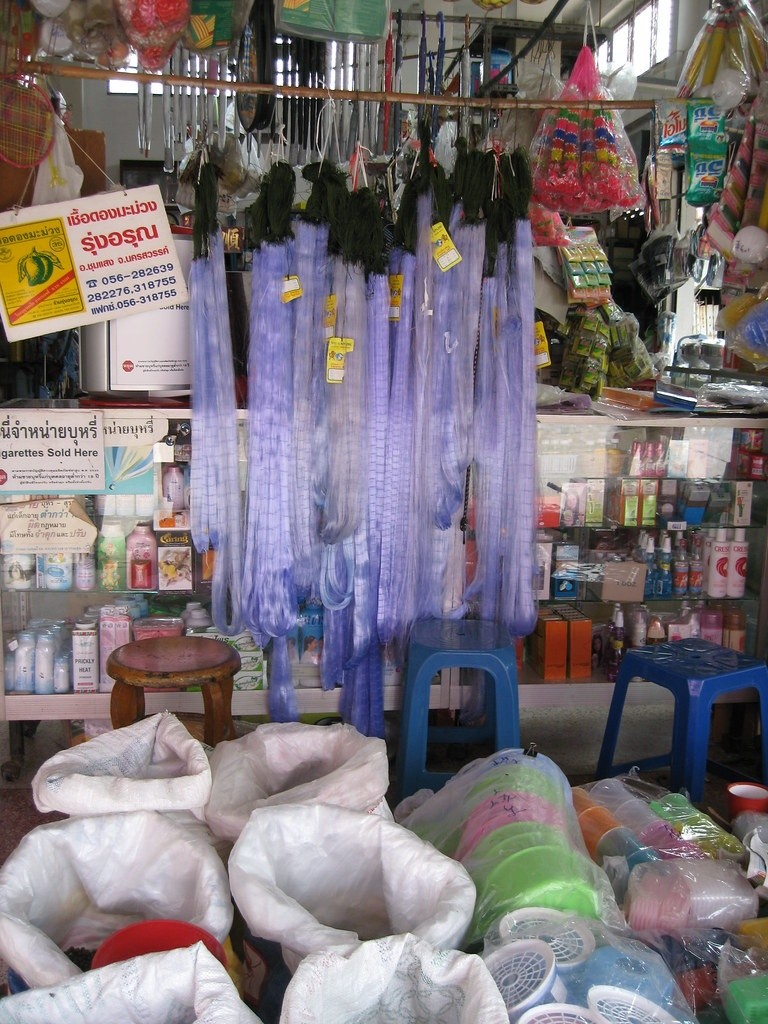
91;920;226;972
413;749;600;940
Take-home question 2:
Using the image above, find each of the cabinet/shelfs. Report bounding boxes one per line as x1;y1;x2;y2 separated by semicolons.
391;13;615;151
451;404;768;723
600;130;651;336
1;398;451;785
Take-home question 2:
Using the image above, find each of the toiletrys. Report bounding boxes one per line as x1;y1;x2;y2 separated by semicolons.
5;618;72;695
591;529;750;597
607;601;747;683
274;598;327;688
2;514;157;591
70;592;267;693
381;634;403;686
162;466;191;509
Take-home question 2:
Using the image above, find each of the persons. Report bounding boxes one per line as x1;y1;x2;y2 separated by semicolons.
560;490;580;526
301;635;323;665
288;636;296;661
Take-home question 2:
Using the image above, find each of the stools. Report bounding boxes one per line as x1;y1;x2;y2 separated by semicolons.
106;637;241;748
400;618;521;797
596;636;768;811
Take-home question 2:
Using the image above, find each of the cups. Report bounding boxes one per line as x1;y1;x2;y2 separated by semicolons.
572;778;744;875
725;781;767;820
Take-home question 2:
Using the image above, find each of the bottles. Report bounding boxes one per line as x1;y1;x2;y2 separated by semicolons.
606;528;749;680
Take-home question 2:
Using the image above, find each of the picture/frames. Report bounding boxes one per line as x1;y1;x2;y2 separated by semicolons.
119;158;177;203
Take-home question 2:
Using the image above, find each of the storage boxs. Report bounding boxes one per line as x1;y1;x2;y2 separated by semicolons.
183;627;269;691
523;602;593;680
156;531;216;595
534;538;579;600
535;477;754;529
666;438;707;477
587;547;649;603
276;0;388;45
